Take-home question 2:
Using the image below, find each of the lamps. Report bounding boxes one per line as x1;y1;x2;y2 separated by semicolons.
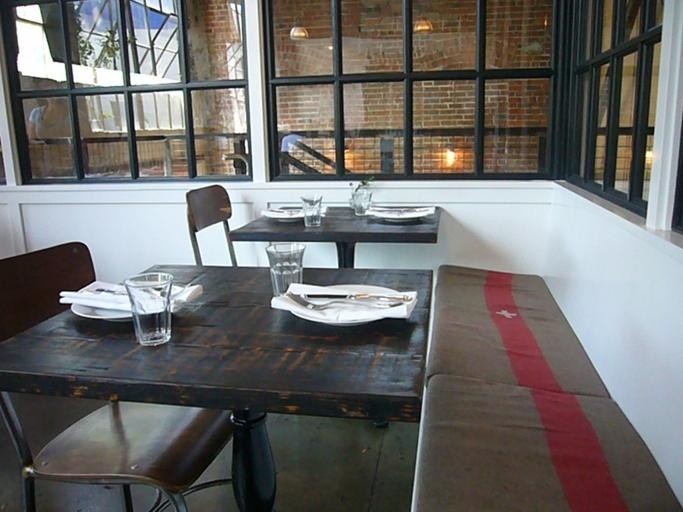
288;0;307;41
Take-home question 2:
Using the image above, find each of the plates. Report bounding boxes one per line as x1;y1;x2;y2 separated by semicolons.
70;304;133;324
370;204;435;222
290;284;401;326
264;207;304;223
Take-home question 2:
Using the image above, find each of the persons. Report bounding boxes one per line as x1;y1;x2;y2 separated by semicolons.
27;74;93;177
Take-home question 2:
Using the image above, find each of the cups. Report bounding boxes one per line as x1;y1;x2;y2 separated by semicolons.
352;183;374;217
265;241;306;297
124;273;173;346
301;194;325;228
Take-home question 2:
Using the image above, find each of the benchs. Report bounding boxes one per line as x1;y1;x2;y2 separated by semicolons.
410;263;682;510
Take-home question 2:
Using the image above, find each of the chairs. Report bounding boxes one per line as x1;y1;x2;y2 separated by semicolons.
0;242;237;509
184;185;238;267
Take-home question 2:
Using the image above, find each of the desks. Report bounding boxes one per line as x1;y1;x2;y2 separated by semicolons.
227;207;441;269
2;265;440;509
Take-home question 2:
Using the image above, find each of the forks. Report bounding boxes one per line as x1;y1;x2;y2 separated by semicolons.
284;292;403;310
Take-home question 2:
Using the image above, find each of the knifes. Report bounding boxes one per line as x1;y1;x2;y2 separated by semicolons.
306;291;411;301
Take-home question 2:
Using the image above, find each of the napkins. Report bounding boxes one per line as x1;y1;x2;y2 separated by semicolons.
271;283;418;321
60;281;203;314
260;208;328;218
364;207;436;218
